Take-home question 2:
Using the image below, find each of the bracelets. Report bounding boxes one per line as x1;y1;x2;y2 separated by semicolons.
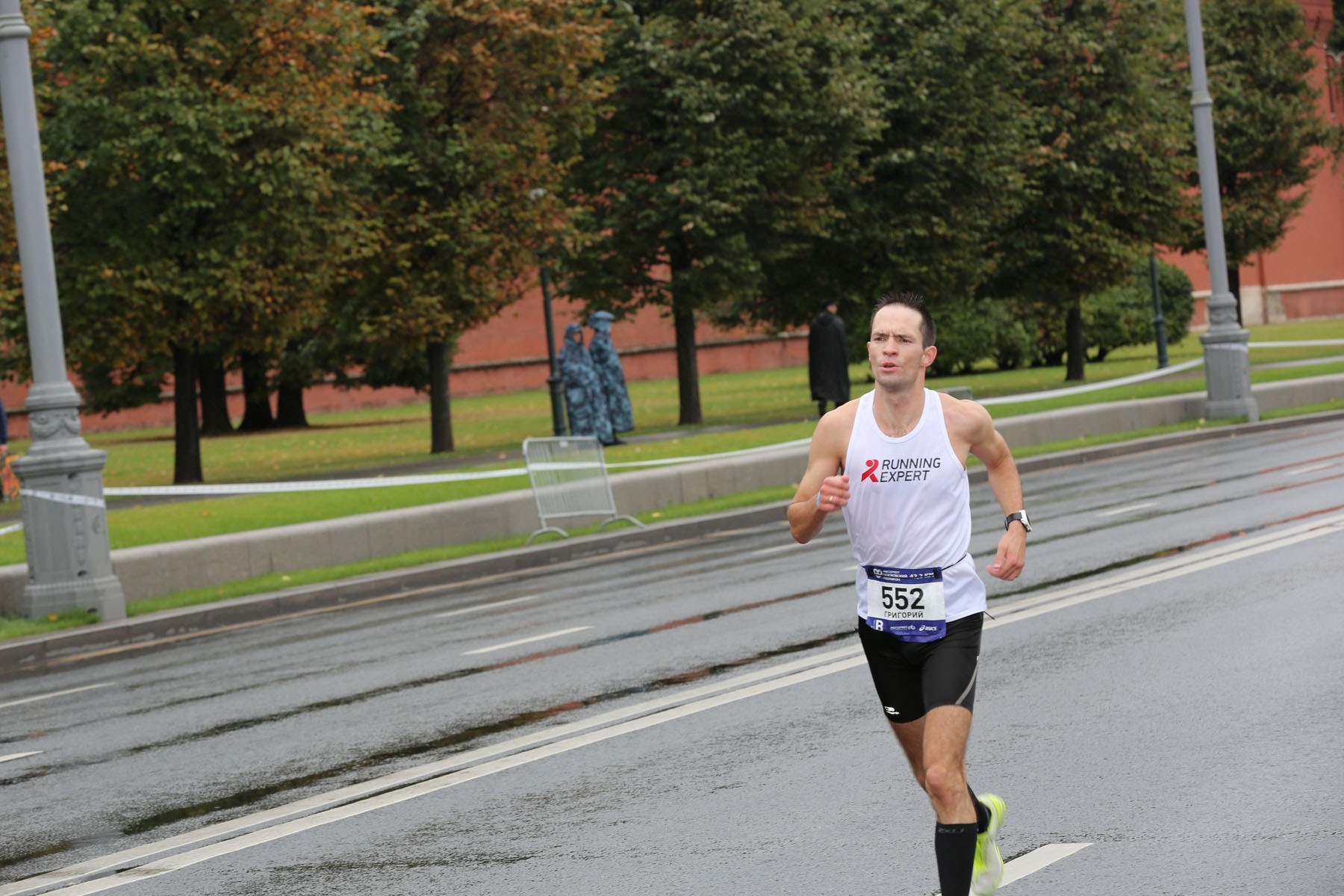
817;492;820;508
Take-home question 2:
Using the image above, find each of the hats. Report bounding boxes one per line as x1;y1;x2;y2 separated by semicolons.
589;311;616;334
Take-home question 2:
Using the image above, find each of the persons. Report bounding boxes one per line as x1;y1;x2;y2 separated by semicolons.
807;301;851;418
556;310;635;446
785;298;1033;896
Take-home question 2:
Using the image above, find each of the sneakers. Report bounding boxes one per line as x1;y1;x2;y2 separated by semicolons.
971;793;1006;896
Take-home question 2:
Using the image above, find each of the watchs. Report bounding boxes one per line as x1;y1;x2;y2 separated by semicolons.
1004;508;1033;533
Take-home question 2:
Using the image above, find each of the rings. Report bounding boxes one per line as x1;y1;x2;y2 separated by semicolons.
828;495;834;505
